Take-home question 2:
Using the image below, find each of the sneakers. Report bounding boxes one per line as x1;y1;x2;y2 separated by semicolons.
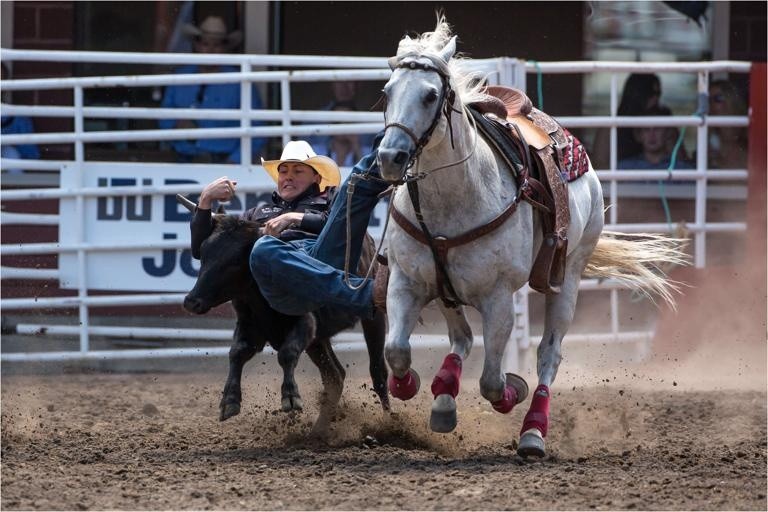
370;250;389;312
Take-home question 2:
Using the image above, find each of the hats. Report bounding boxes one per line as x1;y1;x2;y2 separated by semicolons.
259;141;341;193
188;16;241;45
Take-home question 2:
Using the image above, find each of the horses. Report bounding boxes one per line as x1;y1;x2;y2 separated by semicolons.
375;7;700;460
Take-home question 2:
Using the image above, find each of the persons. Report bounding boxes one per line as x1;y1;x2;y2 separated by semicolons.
190;139;394;317
158;13;269;165
0;60;40;208
297;81;359;145
621;104;693;185
153;2;193;97
311;101;374;166
589;69;686;170
694;77;751;173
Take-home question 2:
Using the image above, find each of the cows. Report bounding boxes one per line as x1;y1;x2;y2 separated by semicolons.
174;192;402;454
